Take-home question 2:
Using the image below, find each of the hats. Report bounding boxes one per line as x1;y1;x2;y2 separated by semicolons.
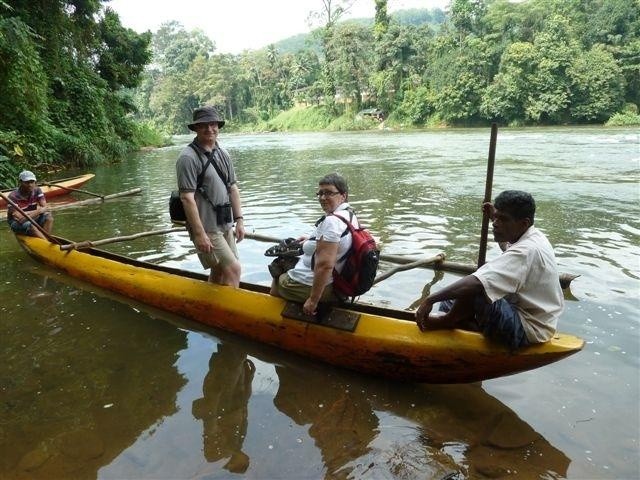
18;170;36;182
187;106;225;130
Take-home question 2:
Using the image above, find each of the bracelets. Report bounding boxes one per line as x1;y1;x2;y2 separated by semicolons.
234;217;243;222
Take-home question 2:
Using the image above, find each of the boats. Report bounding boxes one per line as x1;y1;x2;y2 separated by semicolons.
14;229;586;385
0;174;96;211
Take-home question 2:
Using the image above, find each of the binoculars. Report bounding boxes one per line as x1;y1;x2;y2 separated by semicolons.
216;202;232;226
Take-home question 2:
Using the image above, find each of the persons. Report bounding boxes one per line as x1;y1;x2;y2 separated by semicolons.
7;170;54;240
191;339;254;473
176;106;245;288
270;173;359;316
417;189;565;349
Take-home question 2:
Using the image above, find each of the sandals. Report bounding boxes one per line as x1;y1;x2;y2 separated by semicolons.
265;237;304;256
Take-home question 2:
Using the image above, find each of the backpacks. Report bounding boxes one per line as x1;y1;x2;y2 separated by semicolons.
332;213;379;297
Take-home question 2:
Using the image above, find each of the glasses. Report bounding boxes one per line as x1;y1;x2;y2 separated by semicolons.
316;190;341;196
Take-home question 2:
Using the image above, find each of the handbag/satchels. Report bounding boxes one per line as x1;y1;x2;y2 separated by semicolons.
169;190;186;221
